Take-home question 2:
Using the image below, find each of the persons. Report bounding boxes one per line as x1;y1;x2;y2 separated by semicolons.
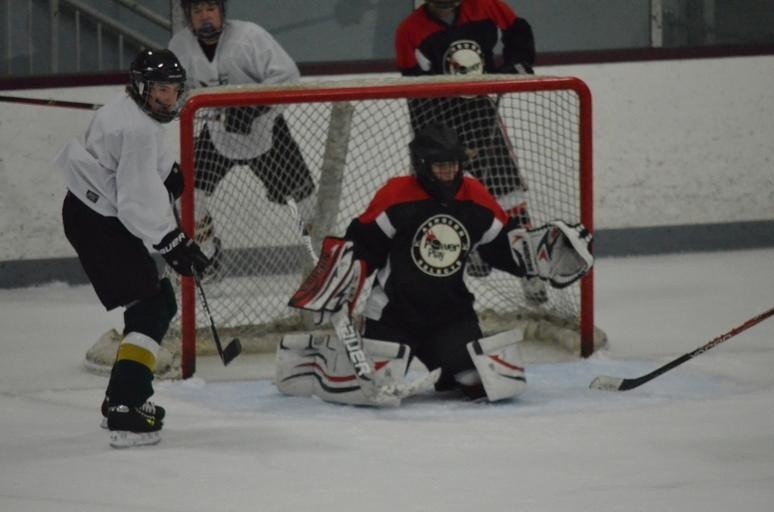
276;121;594;409
394;0;536;230
53;47;213;449
165;2;317;287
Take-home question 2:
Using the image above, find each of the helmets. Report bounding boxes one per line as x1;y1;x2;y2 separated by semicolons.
408;121;469;204
180;0;228;46
124;47;188;124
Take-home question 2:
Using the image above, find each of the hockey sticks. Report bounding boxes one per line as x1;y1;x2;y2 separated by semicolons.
171;191;243;366
590;308;772;391
287;197;442;403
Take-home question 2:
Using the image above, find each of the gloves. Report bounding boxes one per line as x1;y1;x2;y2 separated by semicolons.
152;225;211;278
222;105;272;137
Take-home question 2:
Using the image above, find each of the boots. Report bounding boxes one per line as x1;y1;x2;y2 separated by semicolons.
99;359;167;421
105;358;164;434
